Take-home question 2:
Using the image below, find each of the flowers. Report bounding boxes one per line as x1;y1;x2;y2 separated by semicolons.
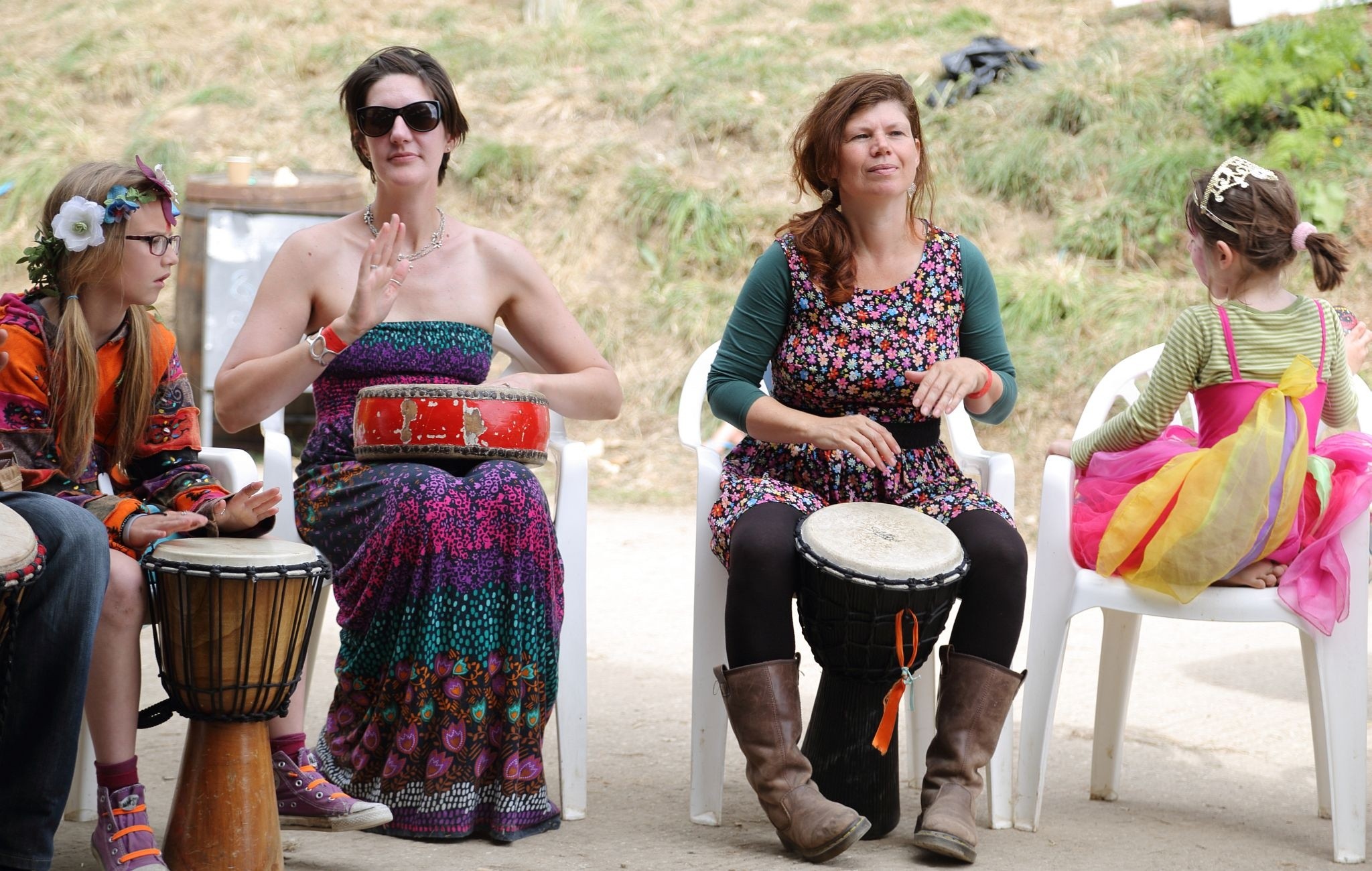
15;154;180;300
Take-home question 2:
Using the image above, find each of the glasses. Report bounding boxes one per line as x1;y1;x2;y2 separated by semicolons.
114;235;181;255
356;101;443;138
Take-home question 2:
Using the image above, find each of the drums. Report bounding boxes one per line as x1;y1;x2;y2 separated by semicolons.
352;383;551;470
0;502;48;650
140;537;332;871
791;501;971;841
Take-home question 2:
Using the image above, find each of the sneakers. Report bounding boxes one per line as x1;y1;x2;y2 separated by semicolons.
91;783;169;871
271;747;394;832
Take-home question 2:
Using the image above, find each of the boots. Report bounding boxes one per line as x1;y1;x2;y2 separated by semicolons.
911;644;1027;862
713;651;872;865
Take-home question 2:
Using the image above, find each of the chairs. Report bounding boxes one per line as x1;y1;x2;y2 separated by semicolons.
1012;341;1372;864
677;338;1012;831
260;323;590;820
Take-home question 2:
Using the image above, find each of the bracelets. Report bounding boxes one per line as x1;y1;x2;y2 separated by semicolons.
298;323;352;367
967;360;993;399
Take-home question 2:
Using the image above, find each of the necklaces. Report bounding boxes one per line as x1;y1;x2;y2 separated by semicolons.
363;202;446;273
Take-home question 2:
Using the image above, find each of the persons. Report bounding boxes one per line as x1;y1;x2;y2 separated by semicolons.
1044;156;1361;590
0;152;284;871
705;72;1028;867
211;45;624;847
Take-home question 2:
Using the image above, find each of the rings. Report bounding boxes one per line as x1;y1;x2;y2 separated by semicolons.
214;509;225;516
180;509;188;516
501;382;511;389
390;278;403;287
370;264;378;269
